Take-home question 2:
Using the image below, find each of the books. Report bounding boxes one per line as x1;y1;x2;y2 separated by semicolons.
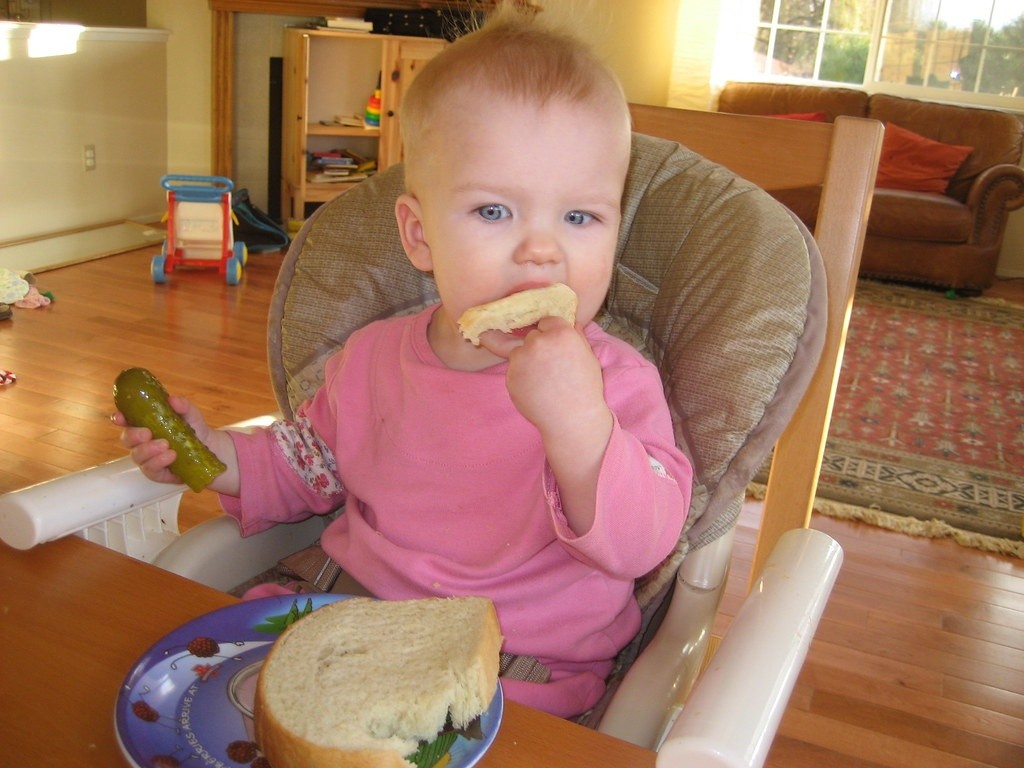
308;149;377;178
307;17;373;33
320;114;364;127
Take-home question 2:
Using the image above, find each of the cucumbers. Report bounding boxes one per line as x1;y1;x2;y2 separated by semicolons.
112;367;228;493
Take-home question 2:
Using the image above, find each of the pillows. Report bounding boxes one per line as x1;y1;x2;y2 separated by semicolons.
875;117;976;193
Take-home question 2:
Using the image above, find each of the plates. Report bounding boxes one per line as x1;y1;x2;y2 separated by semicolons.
112;594;504;768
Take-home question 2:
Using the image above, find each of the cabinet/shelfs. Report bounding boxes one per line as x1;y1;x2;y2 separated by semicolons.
279;23;450;244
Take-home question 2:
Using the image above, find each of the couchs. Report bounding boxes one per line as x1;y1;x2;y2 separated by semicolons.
717;81;1024;298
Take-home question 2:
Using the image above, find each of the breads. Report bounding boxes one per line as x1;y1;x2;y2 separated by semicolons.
456;283;577;346
252;594;504;768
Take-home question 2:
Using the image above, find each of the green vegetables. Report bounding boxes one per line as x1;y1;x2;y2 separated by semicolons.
254;597;485;768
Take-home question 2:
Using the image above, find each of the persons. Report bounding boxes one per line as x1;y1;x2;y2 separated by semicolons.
110;21;693;722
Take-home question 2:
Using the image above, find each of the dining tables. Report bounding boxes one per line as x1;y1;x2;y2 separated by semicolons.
1;528;656;767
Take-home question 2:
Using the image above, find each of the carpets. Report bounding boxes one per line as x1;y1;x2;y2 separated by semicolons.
743;274;1024;564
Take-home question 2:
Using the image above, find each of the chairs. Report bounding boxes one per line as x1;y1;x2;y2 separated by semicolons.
1;100;886;767
